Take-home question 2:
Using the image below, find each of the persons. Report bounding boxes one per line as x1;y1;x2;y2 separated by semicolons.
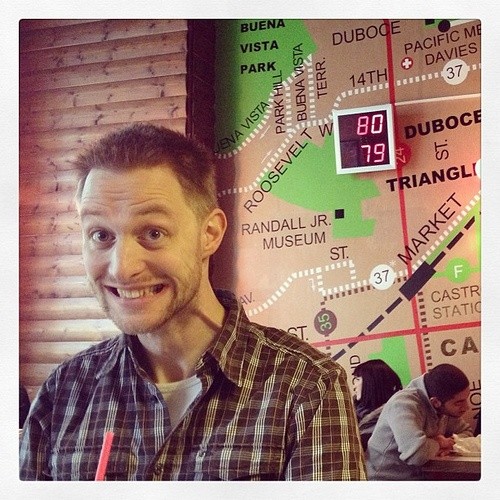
365;363;474;481
351;359;403;454
19;121;368;481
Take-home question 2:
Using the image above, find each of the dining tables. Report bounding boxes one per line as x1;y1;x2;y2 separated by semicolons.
421;454;481;481
